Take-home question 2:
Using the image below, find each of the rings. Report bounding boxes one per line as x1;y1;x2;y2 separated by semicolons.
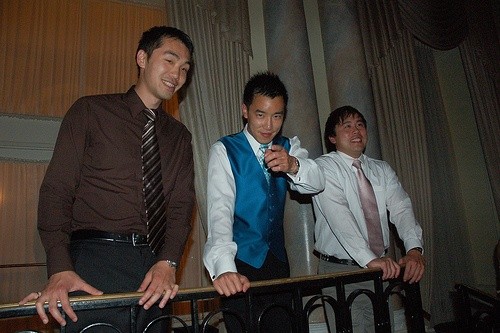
36;291;40;300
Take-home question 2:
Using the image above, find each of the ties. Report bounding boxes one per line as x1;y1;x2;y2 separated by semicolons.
259;144;270;180
352;160;385;257
141;108;168;256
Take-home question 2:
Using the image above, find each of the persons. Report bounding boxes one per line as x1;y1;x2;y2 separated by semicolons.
202;71;325;333
310;106;424;333
17;26;196;333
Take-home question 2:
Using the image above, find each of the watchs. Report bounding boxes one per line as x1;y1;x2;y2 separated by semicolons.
164;259;177;269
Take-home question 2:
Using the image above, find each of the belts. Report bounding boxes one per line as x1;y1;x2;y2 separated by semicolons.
313;249;358;266
71;229;148;246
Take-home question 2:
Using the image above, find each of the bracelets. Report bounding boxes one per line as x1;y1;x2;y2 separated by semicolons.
291;157;301;176
408;246;422;254
